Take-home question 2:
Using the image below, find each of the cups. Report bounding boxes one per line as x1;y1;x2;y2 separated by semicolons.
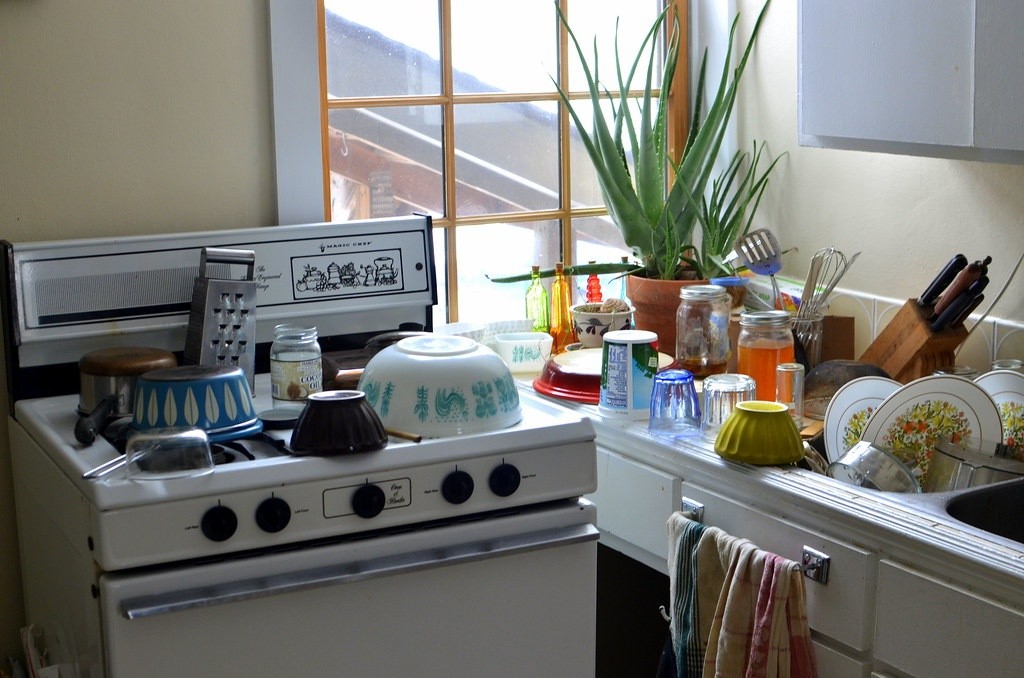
597;330;660;422
701;374;756;444
775;362;804;432
646;368;701;439
932;359;1024;380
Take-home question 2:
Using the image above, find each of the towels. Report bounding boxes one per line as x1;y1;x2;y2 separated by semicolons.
665;509;819;678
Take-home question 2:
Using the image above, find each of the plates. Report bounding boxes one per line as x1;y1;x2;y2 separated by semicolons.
823;369;1024;489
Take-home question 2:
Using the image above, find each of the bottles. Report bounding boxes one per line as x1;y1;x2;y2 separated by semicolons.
525;257;629;356
738;310;794;418
270;321;322;411
709;276;749;374
676;285;730;382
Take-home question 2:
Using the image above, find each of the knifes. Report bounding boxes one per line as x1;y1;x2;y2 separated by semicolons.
914;253;993;333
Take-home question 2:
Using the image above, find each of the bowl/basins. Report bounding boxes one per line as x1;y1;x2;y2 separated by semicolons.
432;313;535;344
714;400;805;467
125;427;216;480
290;390;389;456
533;349;680;406
355;335;522;440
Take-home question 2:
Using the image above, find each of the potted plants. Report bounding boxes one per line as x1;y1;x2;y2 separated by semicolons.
485;0;791;357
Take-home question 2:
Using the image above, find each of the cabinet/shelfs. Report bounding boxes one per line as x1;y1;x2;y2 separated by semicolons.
586;443;1024;678
798;0;1024;166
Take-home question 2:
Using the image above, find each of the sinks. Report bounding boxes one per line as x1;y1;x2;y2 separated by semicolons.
787;420;1023;494
944;478;1024;545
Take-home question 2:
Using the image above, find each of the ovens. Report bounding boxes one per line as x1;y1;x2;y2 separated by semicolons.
10;505;604;678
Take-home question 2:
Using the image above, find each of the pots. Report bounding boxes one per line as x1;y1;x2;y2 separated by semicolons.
74;346;177;445
81;365;264;480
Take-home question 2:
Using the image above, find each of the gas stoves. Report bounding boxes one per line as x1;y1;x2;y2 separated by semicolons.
0;210;599;572
